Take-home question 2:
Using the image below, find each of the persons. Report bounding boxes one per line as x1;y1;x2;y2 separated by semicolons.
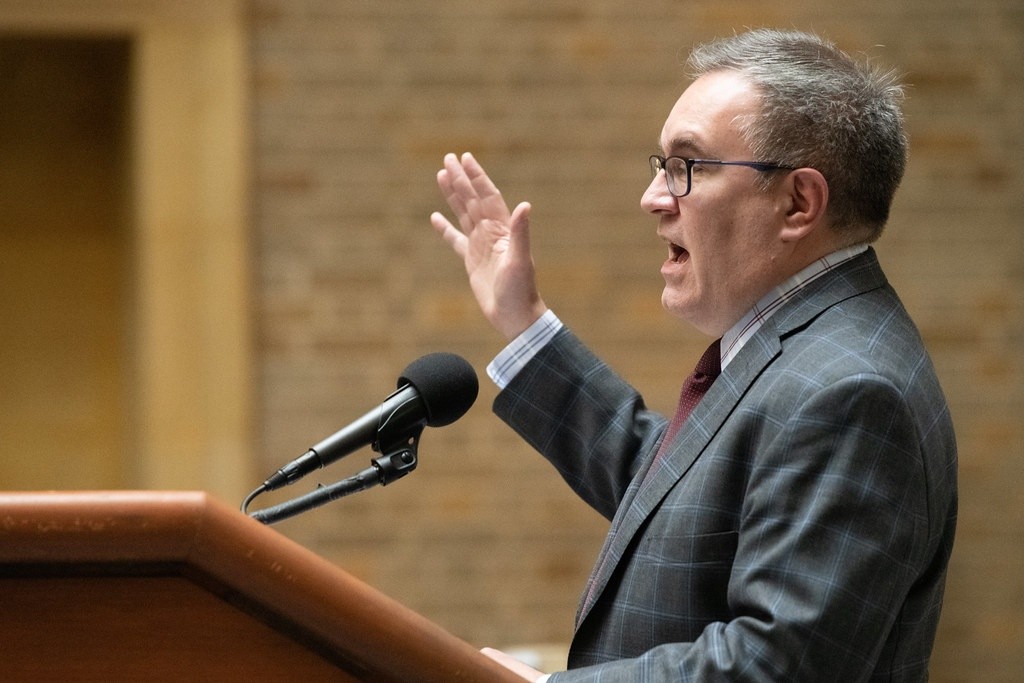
426;26;958;683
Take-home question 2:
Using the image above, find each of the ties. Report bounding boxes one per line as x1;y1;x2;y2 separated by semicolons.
650;334;721;470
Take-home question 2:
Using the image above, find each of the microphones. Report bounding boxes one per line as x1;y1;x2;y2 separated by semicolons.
263;351;480;494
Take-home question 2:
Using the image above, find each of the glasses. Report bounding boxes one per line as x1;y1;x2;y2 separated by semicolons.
647;155;799;198
